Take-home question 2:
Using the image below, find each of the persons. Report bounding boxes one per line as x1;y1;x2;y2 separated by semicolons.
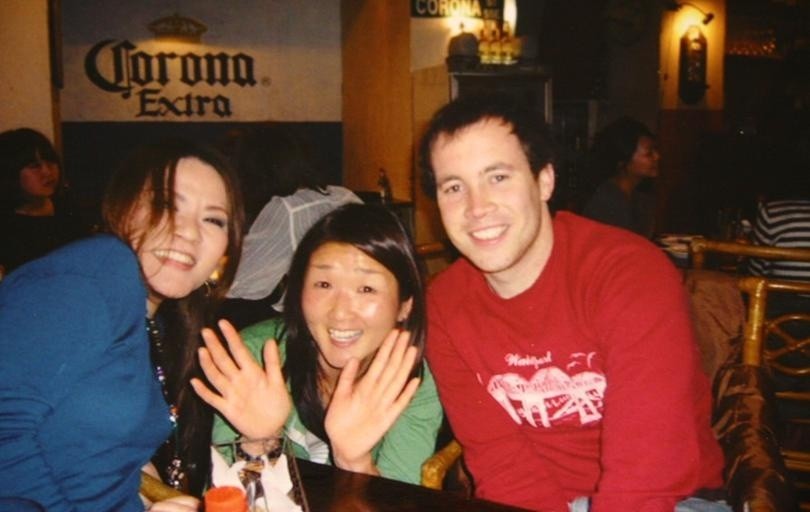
1;129;247;512
743;167;810;369
415;91;734;512
0;128;87;281
187;203;445;512
223;118;365;314
579;124;661;236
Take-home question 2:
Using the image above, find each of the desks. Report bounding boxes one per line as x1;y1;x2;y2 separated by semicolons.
199;449;531;512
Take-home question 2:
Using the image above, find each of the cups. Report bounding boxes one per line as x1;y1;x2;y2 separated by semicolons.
199;484;250;512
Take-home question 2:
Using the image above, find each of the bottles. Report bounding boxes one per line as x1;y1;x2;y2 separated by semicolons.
476;20;515;64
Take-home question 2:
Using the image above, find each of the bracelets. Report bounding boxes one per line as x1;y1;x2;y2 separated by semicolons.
235;447;281;461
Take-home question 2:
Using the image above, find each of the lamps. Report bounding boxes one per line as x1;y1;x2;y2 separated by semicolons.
678;2;713;25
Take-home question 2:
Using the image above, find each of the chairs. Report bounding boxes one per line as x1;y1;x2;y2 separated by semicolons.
419;265;771;511
412;235;464;282
689;237;808;474
